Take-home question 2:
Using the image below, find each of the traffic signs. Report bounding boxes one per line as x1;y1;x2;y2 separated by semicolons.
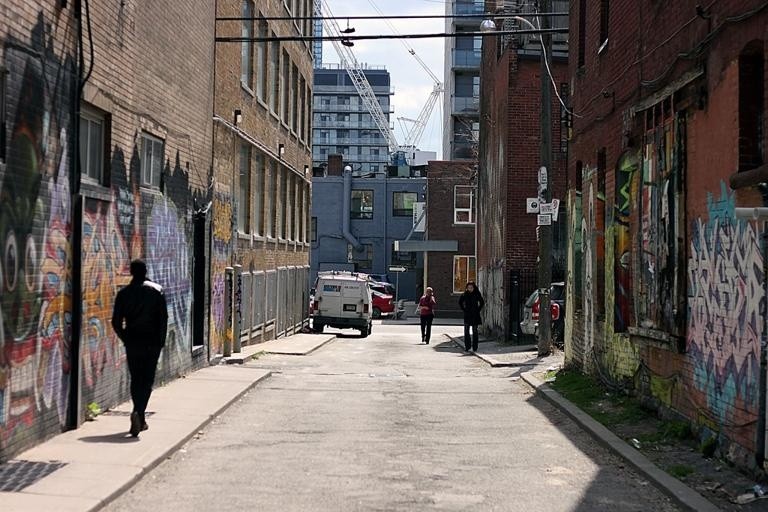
387;265;408;273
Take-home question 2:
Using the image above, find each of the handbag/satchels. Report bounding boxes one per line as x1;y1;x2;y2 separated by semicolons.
420;307;430;315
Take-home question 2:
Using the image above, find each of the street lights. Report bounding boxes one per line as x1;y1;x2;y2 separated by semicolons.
478;15;554;356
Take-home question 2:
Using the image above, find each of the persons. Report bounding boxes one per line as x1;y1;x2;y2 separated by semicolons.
417;286;438;345
110;259;169;438
458;281;485;353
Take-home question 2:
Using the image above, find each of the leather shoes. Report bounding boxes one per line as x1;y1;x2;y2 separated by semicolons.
130;413;148;437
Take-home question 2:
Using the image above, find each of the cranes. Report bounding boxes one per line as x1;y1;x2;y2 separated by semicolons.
310;0;444;178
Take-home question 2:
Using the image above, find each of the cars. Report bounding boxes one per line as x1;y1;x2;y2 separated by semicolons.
309;270;395;338
519;282;565;350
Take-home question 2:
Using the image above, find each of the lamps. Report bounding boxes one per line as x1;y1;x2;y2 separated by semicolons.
278;143;285;159
234;108;242;127
304;165;309;175
479;16;590;119
